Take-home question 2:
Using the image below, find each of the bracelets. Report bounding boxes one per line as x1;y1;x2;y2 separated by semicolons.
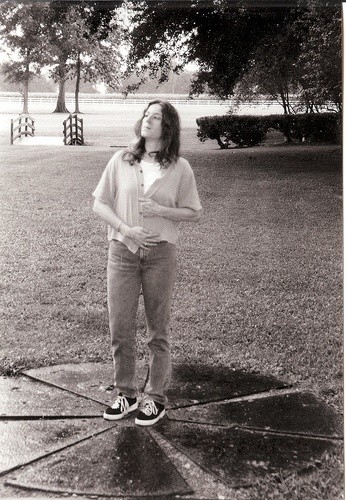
117;221;122;232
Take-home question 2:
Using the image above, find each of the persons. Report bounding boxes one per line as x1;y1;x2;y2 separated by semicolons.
90;99;205;426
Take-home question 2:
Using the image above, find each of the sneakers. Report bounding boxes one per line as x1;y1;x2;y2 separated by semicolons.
103;393;137;420
134;399;165;426
